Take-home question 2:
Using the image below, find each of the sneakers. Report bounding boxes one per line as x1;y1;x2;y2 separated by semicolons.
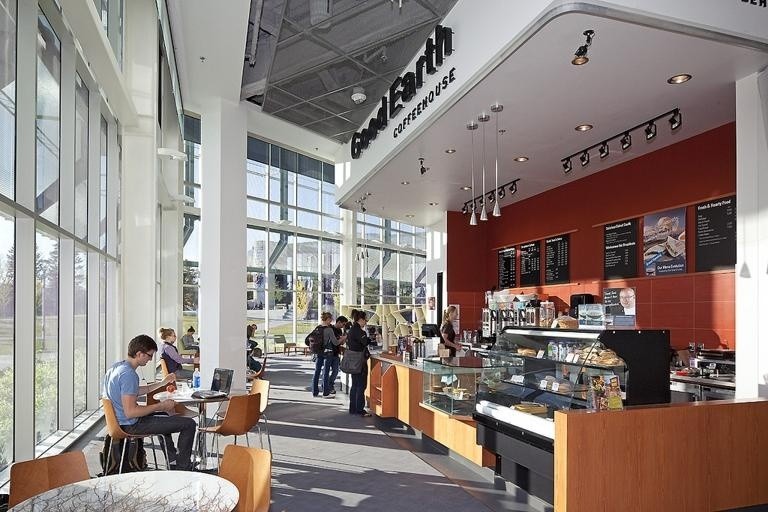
312;385;372;418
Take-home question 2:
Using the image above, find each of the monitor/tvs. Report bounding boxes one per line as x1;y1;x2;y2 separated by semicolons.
422;324;445;343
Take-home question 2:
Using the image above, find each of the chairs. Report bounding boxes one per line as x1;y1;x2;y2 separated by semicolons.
217;444;272;511
102;335;273;479
272;334;297;355
8;450;92;511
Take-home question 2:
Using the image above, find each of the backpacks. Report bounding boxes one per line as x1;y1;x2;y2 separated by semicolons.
307;325;328;354
95;433;148;478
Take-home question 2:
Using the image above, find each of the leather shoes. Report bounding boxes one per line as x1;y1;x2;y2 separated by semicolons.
167;460;200;472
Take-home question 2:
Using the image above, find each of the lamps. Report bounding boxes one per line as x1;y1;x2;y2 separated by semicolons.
359;195;367;262
559;102;684;182
354;197;361;264
418;156;428;178
460;176;520;218
488;103;506;218
475;112;495;222
363;191;373;259
568;26;598;70
464;118;482;229
359;198;367;213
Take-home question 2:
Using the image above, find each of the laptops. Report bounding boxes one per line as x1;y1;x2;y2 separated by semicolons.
191;368;234;399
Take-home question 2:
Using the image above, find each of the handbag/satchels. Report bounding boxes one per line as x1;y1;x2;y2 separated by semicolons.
339;349;367;374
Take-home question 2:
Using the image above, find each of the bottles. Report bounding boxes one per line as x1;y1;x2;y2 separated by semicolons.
539;301;555;327
459;329;481;343
192;367;201;389
412;338;425;359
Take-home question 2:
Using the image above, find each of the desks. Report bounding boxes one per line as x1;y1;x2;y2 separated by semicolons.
6;471;239;511
288;343;311;357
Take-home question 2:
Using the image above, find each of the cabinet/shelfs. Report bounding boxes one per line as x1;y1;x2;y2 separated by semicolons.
471;321;675;509
415;349;524;472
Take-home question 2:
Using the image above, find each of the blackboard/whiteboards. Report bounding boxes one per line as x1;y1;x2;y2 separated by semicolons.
695;195;736;272
604;218;638;280
498;233;570;289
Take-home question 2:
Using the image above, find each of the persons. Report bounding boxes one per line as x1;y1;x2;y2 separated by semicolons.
310;311;347;399
246;324;263;379
440;305;461;357
320;316;349;396
182;327;200;352
345;308;372;418
103;335;195;470
618;288;635;316
159;327;198;379
343;309;358;336
